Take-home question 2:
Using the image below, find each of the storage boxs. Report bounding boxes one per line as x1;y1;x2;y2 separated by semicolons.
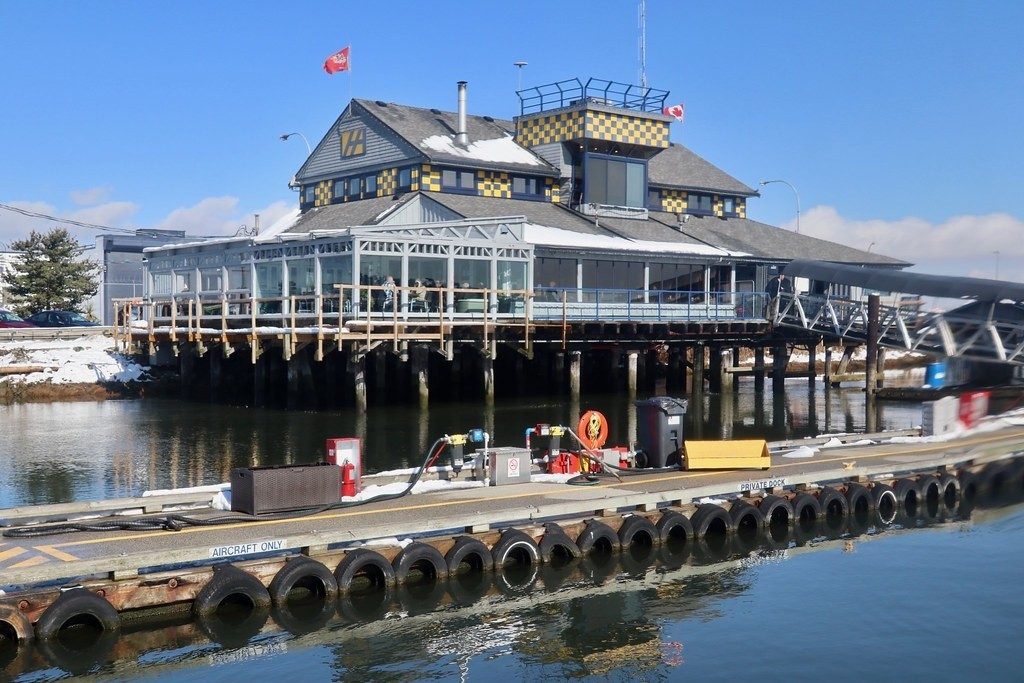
230;463;342;518
475;447;532;486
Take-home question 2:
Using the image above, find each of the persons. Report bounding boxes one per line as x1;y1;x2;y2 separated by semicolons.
408;279;426;303
546;282;560;301
380;275;398;312
765;273;784;319
428;277;474;310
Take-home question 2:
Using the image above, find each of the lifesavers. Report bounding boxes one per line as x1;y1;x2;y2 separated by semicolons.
618;512;658;547
271;555;338;603
540;525;583;563
658;510;694;546
444;533;494;576
37;589;119;639
578;412;608;447
578;520;619;553
694;473;981;540
1;604;35;642
394;542;447;584
196;564;272;616
335;548;397;595
494;529;541;567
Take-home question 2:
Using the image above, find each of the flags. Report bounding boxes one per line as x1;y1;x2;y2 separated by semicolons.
664;103;683;122
323;46;349;74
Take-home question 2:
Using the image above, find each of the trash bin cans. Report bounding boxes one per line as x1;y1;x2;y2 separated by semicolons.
631;395;687;468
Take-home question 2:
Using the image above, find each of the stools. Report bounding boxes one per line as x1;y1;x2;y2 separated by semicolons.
410;298;428;312
343;299;350;312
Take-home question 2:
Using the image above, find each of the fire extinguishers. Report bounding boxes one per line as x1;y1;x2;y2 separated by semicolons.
341;457;357;496
